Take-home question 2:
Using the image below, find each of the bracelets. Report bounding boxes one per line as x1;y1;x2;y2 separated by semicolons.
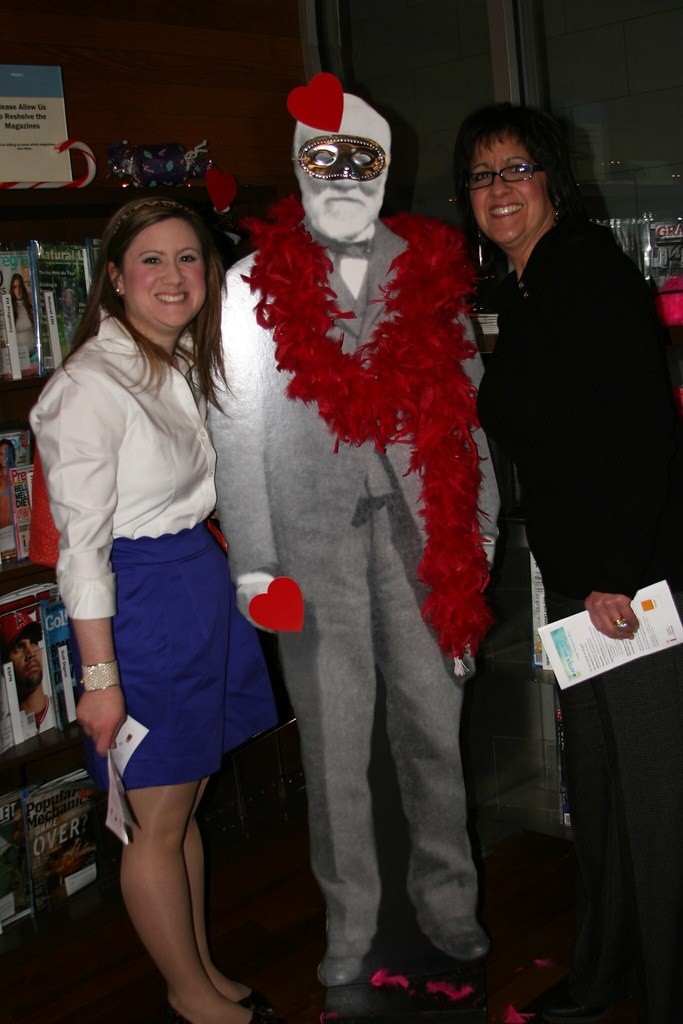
80;660;120;691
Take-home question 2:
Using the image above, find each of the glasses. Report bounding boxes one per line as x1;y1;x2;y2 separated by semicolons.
465;163;544;190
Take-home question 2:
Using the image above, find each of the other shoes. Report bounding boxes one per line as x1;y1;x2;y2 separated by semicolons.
317;933;377;988
236;980;276;1015
426;914;487;959
166;996;286;1024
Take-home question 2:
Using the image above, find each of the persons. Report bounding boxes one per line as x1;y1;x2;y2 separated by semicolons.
212;91;502;985
60;278;82;353
0;612;54;737
28;196;276;1024
0;438;16;527
10;273;35;346
453;104;683;1024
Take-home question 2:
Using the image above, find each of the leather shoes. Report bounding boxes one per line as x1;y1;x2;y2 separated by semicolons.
520;969;636;1024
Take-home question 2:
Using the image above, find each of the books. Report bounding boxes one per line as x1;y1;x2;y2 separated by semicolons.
0;238;117;937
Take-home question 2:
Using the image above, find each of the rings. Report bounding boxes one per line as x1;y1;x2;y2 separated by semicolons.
614;616;624;626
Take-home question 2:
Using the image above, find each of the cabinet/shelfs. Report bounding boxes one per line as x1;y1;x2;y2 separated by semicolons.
0;177;281;931
